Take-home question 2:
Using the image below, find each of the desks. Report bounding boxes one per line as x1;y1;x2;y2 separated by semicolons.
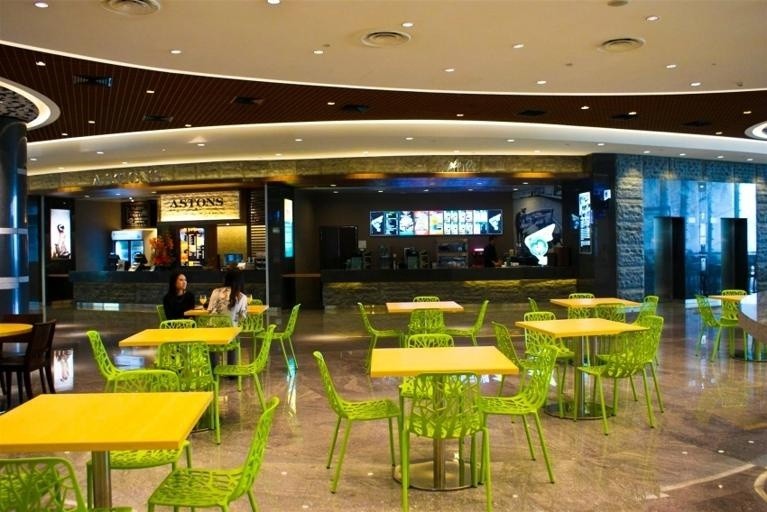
1;391;214;510
369;347;518;491
708;295;763;361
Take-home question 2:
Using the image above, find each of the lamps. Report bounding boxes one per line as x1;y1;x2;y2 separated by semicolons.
721;290;752;360
693;295;748;362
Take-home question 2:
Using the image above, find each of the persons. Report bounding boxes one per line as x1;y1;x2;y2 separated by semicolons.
162;271;197;328
483;235;501;267
207;268;248;381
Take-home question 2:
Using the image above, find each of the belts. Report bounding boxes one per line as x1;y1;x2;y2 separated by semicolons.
200;294;207;305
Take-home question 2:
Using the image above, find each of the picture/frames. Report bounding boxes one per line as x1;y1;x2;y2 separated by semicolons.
708;295;763;361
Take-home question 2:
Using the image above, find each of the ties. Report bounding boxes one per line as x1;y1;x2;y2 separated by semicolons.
134;254;148;264
224;253;243;265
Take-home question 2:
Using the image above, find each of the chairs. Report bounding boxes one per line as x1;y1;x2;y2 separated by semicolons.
313;351;411;494
2;452;62;511
1;457;133;511
398;334;465;452
721;290;752;360
147;397;281;510
86;370;195;510
470;345;558;484
693;295;748;362
491;293;665;436
397;372;490;511
358;288;491;374
0;297;305;443
